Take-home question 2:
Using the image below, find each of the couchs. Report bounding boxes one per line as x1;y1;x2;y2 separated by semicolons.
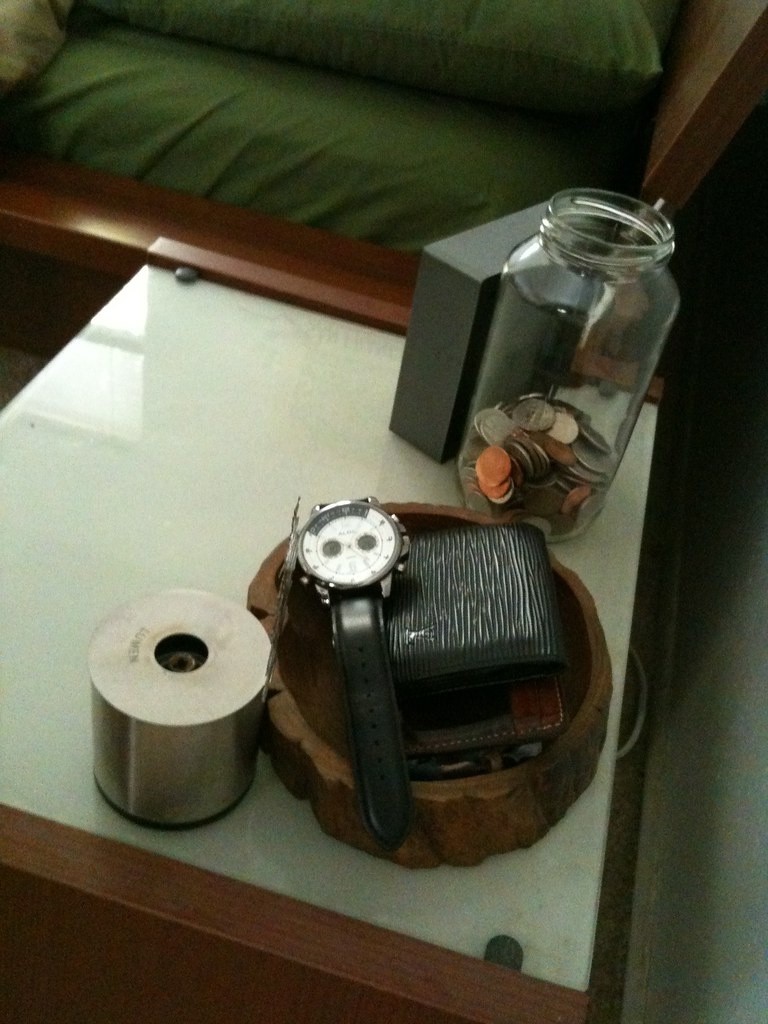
0;0;767;356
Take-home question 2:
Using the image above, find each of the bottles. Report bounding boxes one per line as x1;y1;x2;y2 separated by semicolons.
456;187;681;544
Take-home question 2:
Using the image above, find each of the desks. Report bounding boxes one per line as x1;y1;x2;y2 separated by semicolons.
0;236;666;1024
